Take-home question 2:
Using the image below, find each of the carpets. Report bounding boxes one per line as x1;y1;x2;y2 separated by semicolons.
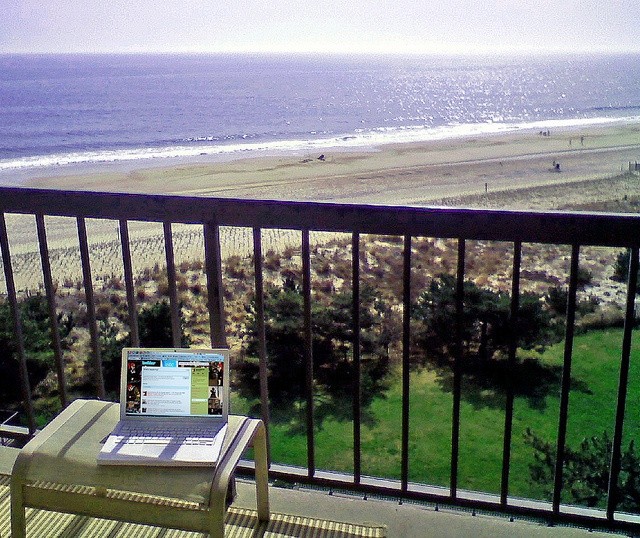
0;472;386;537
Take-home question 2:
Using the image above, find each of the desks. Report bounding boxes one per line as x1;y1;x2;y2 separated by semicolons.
10;399;269;538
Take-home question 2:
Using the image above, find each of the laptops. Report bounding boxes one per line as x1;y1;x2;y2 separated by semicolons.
97;346;231;468
